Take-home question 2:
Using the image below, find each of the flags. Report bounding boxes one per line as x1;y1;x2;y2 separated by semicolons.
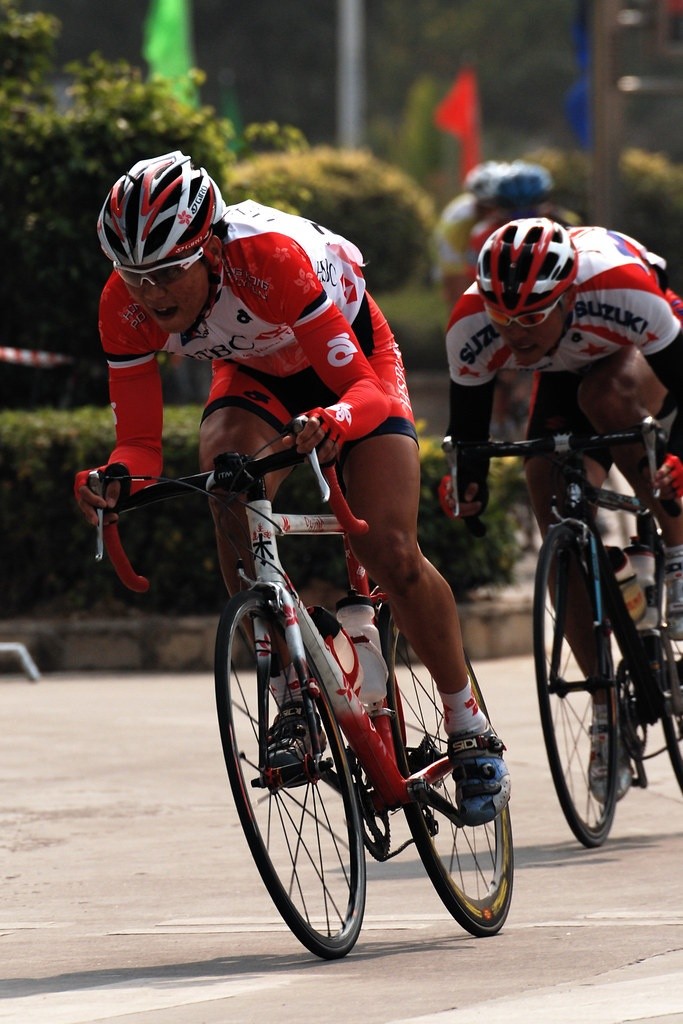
141;0;199;105
433;64;481;183
561;0;593;154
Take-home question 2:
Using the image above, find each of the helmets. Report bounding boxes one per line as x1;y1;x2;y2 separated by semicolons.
493;163;554;206
476;217;578;315
97;150;228;268
466;162;508;201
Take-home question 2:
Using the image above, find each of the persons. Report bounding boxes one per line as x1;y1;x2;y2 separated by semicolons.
438;159;583;447
436;217;683;803
74;149;511;827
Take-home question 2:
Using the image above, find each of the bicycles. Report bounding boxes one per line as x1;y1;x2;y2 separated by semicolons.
437;413;683;852
78;411;515;960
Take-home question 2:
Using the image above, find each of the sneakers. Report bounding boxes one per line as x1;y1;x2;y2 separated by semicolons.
446;719;511;827
588;717;631;803
663;555;683;640
266;700;326;769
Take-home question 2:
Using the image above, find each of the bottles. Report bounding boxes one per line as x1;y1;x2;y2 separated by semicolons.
306;604;365;695
626;535;660;630
334;589;390;705
602;545;648;622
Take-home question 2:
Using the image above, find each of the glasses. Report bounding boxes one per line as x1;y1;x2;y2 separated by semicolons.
113;230;213;288
483;292;564;328
